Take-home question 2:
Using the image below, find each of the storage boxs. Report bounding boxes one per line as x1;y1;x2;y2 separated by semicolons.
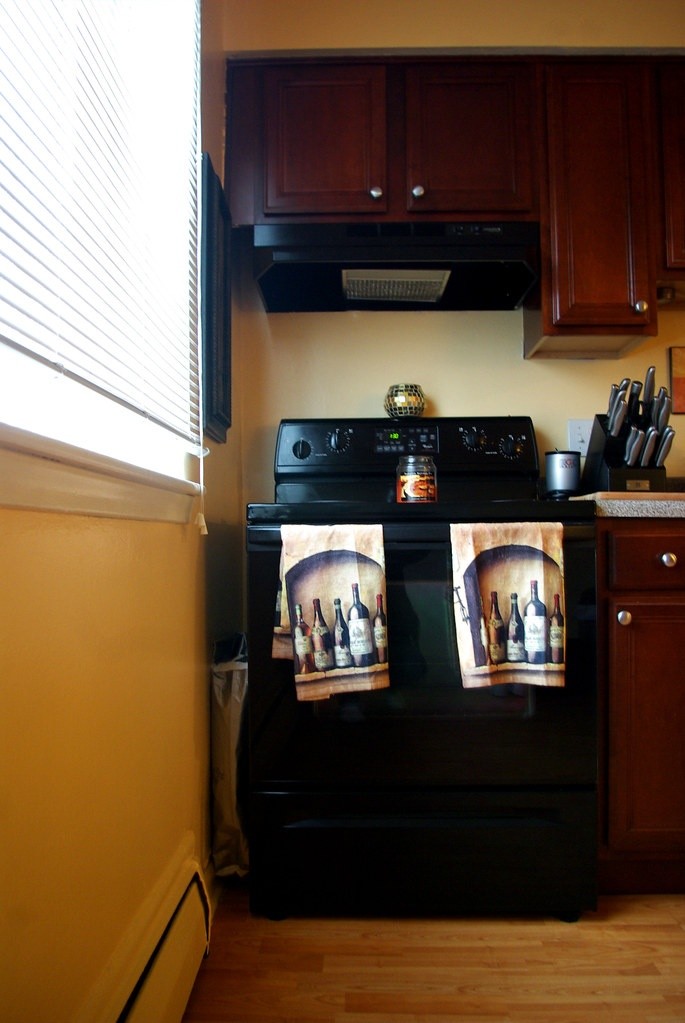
582;414;667;493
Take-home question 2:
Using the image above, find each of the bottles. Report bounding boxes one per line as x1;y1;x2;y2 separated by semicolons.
293;583;387;674
487;580;564;665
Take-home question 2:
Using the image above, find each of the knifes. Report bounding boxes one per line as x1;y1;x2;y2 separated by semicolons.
607;367;676;468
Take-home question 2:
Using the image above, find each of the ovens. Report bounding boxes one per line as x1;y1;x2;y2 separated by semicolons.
247;526;602;914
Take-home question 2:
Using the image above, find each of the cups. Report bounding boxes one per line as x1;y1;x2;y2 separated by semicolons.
545;451;581;495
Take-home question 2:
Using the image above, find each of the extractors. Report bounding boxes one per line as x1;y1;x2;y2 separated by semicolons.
251;222;539;312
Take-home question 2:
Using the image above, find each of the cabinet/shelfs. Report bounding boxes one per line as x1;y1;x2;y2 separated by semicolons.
570;490;685;894
223;54;685;360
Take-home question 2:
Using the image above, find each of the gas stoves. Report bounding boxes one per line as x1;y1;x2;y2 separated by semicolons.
244;417;597;526
